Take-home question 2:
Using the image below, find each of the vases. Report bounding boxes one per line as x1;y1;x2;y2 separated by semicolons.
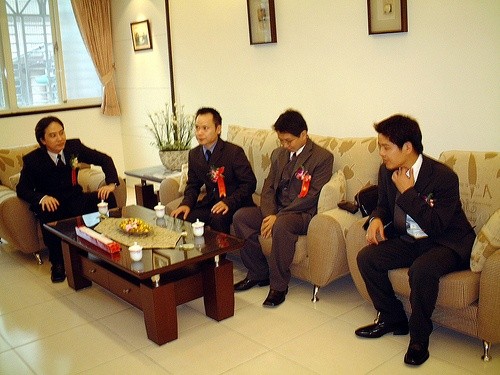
159;150;189;172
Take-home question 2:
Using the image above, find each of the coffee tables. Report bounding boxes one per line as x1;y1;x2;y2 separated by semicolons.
43;204;249;346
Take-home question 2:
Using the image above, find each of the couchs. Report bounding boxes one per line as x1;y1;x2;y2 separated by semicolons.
0;139;128;266
346;150;500;362
158;123;385;302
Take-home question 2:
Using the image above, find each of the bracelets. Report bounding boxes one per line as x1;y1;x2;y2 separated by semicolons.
114;183;117;187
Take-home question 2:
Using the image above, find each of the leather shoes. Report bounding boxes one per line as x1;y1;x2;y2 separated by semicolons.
263;287;288;307
51;264;65;282
355;318;409;338
234;277;270;290
404;337;429;365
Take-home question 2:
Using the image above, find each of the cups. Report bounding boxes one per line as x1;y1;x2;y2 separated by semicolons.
97;199;108;214
128;242;143;261
154;202;165;218
191;218;205;237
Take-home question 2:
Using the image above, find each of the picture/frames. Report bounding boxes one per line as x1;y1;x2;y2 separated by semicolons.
130;21;153;52
247;0;277;46
367;0;408;36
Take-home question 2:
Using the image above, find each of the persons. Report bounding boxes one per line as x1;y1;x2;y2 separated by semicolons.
16;116;121;283
171;108;257;258
355;114;476;366
233;110;334;307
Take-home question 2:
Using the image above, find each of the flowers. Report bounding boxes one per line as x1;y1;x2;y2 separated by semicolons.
70;156;79;169
145;102;197;152
294;164;311;182
417;192;435;208
207;164;225;182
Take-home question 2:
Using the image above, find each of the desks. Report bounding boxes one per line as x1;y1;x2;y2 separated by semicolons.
124;166;183;182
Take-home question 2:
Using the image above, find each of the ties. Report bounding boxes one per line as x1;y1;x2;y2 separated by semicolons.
394;191;406;234
57;154;65;169
292;153;297;162
206;150;212;164
